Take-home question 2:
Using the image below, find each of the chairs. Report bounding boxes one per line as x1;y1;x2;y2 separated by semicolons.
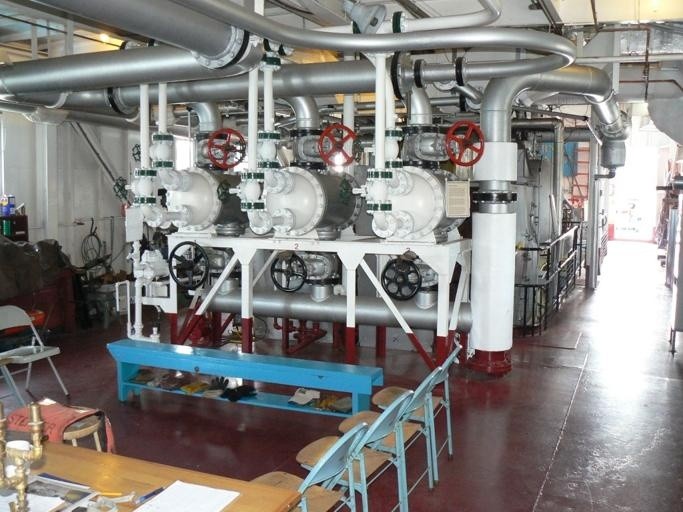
0;305;69;407
249;342;462;512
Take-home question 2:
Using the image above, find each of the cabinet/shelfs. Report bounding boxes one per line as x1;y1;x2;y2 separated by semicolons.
0;271;76;346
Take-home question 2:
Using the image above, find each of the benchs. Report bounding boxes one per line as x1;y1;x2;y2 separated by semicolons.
105;339;385;417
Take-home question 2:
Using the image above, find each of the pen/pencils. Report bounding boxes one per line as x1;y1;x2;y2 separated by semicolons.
135;487;164;504
99;492;123;496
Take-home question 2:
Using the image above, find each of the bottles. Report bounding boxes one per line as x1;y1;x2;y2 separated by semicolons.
0;194;16;216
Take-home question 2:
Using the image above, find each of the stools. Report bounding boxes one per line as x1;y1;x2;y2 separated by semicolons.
10;398;104;451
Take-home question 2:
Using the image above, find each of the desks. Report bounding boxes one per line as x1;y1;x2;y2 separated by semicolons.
0;436;302;512
